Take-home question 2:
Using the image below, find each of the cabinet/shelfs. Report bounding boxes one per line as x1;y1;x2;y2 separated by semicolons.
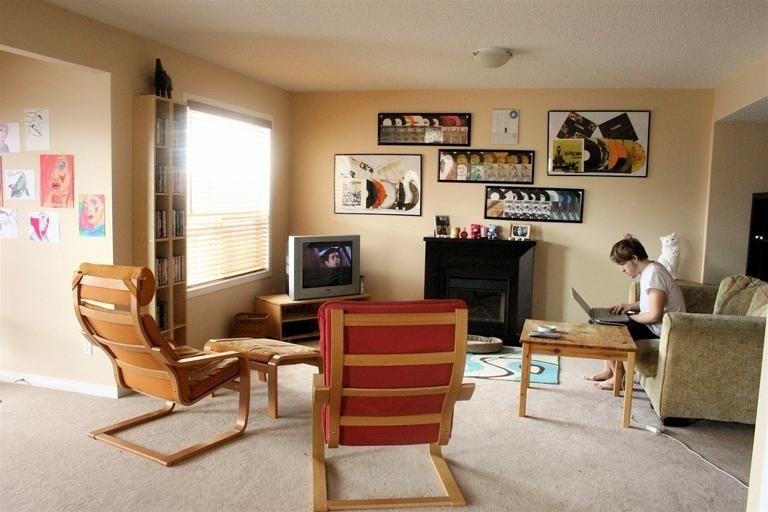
420;236;536;345
255;292;371;342
130;94;187;348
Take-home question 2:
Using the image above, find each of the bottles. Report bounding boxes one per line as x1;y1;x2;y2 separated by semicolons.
450;221;498;241
359;275;365;295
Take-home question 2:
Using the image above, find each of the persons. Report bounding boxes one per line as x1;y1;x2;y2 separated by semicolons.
156;119;185;332
586;236;686;389
323;248;341;269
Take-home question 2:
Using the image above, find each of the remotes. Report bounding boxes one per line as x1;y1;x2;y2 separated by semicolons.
528;331;561;339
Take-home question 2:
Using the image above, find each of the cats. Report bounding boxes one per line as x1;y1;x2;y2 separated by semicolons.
658;232;681;280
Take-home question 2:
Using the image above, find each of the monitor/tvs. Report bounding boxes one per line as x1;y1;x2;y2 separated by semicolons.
285;235;360;301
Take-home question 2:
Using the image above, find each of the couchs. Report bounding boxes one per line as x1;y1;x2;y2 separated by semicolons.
628;275;767;427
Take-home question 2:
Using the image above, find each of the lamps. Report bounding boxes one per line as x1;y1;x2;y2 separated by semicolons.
472;44;512;68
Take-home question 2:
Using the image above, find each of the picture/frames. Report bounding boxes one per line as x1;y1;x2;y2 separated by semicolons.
333;153;423;217
436;149;535;184
433;215;450;237
377;112;471;146
507;223;532;241
483;185;584;223
545;109;649;177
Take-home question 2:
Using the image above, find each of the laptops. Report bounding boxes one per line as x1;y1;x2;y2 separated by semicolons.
571;286;630;327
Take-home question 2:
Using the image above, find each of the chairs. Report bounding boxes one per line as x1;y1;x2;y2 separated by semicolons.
309;298;474;511
70;262;252;467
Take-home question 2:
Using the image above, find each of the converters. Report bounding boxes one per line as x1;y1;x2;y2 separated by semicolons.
646;425;658;432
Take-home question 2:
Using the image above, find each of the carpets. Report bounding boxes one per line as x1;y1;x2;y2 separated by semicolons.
461;345;561;384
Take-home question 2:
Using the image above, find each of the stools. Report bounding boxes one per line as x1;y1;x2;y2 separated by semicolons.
204;336;322;418
